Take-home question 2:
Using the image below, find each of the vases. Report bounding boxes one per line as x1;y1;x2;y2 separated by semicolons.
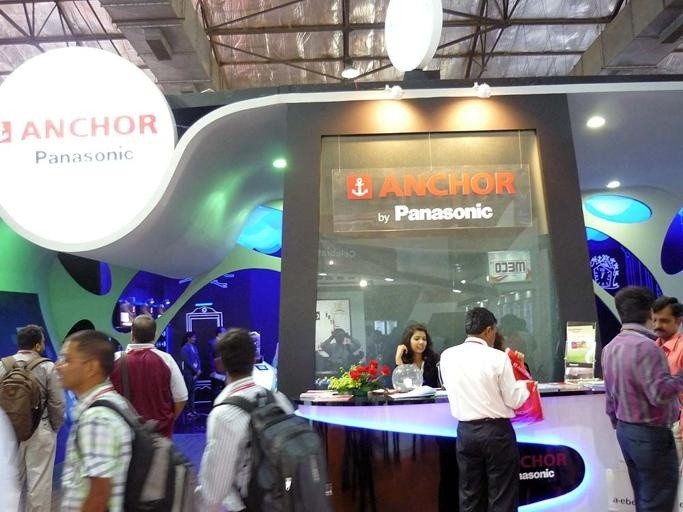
352;388;367;397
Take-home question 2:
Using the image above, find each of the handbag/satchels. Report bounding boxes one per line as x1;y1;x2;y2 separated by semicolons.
508;382;544;423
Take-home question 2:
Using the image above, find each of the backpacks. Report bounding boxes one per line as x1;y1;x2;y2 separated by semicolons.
213;388;333;512
0;356;52;440
74;400;197;512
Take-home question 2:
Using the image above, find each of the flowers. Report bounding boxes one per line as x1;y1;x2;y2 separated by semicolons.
348;358;391;391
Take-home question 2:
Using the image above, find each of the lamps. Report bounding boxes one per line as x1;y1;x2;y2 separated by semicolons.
384;84;403;99
472;81;490;99
341;55;360;79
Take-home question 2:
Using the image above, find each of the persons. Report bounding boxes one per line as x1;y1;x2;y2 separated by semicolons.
649;296;683;512
498;314;537;377
321;328;361;369
601;285;683;512
395;322;440;388
439;307;530;512
